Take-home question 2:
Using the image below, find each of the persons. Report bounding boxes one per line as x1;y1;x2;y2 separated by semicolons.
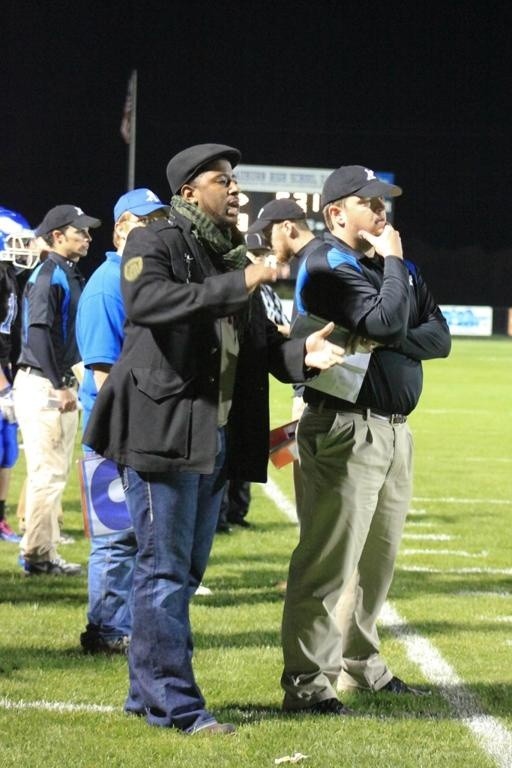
214;196;322;536
12;205;102;576
82;142;345;737
71;188;170;655
280;167;452;715
1;207;38;545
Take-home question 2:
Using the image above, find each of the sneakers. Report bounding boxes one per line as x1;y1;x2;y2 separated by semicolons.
19;553;81;576
380;676;433;697
192;722;237;735
303;697;353;715
1;518;23;544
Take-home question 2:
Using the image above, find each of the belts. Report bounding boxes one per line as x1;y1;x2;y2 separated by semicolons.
309;401;408;425
19;364;76;386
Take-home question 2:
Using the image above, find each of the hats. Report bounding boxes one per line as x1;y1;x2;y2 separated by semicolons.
43;204;101;234
321;165;403;208
166;143;241;194
243;198;305;250
113;187;170;222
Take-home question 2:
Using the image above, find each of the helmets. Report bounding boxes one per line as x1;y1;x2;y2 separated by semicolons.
0;207;40;274
